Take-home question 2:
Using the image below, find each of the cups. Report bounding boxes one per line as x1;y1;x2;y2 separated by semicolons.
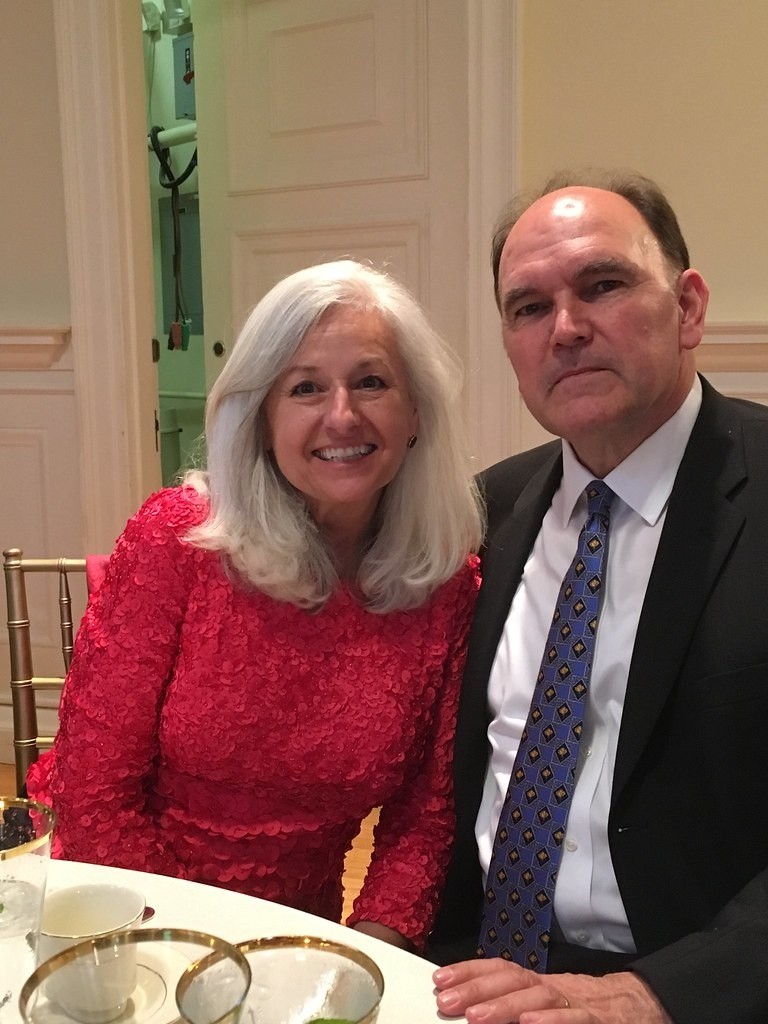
35;883;146;1024
0;798;53;1024
19;927;385;1024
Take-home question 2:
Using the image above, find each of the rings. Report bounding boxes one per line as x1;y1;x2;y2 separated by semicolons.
560;993;571;1009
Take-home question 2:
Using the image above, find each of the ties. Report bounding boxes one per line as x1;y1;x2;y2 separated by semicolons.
475;481;614;975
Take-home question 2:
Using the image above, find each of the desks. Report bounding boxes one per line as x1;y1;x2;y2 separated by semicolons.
0;853;477;1024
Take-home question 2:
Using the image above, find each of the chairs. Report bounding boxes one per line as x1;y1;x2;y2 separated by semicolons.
2;548;123;818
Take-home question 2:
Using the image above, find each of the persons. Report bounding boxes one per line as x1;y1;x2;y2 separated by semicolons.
26;260;483;951
431;166;768;1023
